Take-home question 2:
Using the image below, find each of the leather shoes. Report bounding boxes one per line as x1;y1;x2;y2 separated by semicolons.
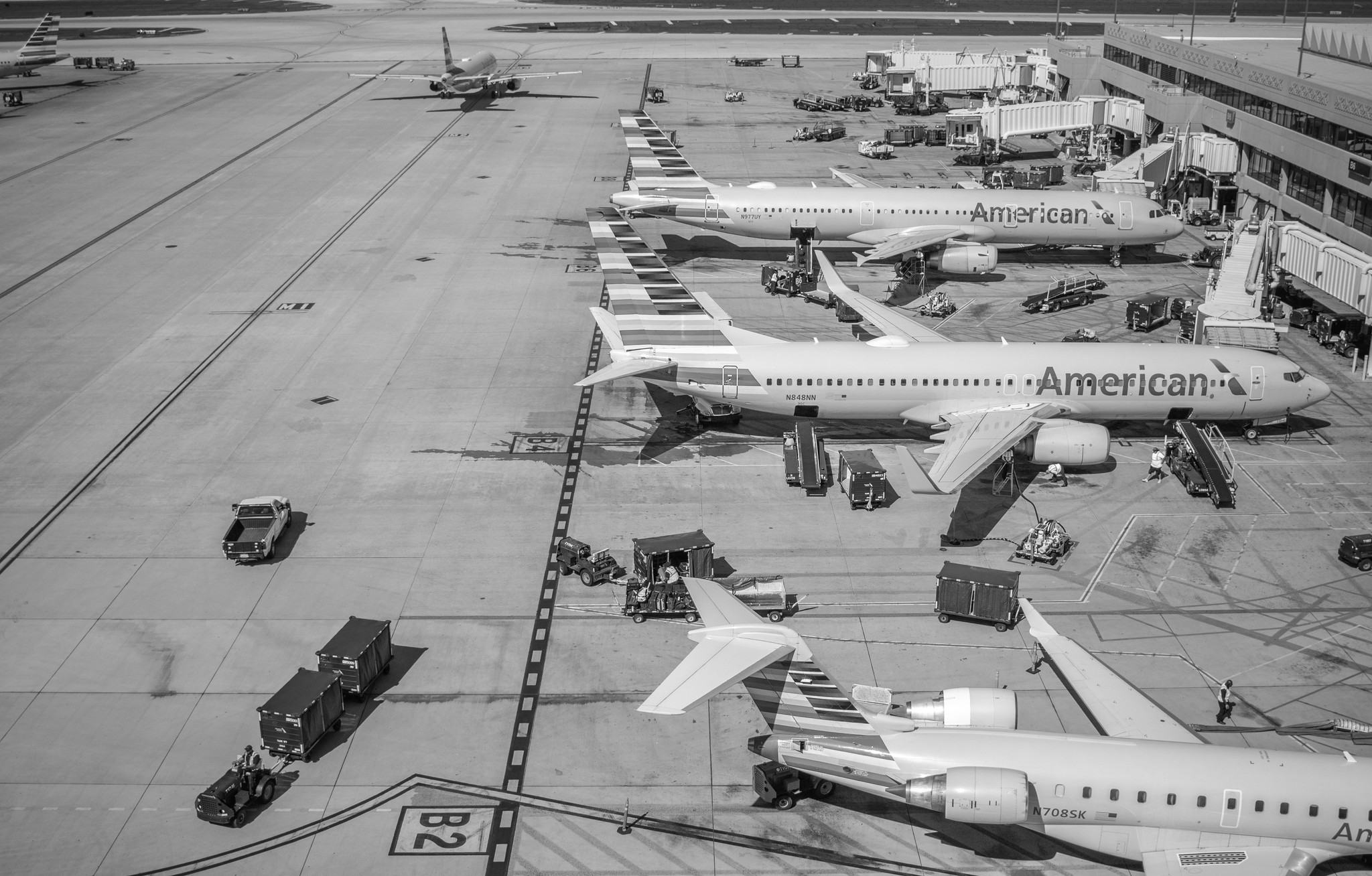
1051;480;1057;483
1061;484;1067;487
1217;721;1225;724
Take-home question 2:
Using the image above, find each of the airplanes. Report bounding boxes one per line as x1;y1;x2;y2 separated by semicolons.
0;12;72;78
347;25;582;100
604;107;1184;276
637;577;1372;876
572;207;1334;496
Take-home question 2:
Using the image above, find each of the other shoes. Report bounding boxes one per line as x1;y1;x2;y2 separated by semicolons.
249;792;253;797
771;293;775;296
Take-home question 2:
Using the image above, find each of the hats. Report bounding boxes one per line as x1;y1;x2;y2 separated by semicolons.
1152;447;1157;451
244;745;252;751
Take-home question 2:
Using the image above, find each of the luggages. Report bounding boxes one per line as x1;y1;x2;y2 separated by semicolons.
627;578;696;611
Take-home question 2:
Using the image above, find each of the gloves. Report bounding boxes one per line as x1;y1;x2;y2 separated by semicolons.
1226;704;1229;709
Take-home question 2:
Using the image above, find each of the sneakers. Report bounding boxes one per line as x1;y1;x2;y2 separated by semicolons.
1142;479;1148;482
1158;479;1161;483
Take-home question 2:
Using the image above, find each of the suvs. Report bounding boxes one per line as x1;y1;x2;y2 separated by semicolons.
1337;533;1372;571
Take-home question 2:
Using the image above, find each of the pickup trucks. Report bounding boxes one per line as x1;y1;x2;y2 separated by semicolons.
222;495;292;563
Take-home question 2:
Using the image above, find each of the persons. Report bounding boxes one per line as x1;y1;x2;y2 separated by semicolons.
771;271;780;296
232;745;260;798
1046;463;1068;487
1142;447;1166;483
1216;680;1233;724
663;562;681;585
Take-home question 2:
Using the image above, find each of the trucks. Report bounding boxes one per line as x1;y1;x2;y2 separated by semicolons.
1203;217;1244;241
